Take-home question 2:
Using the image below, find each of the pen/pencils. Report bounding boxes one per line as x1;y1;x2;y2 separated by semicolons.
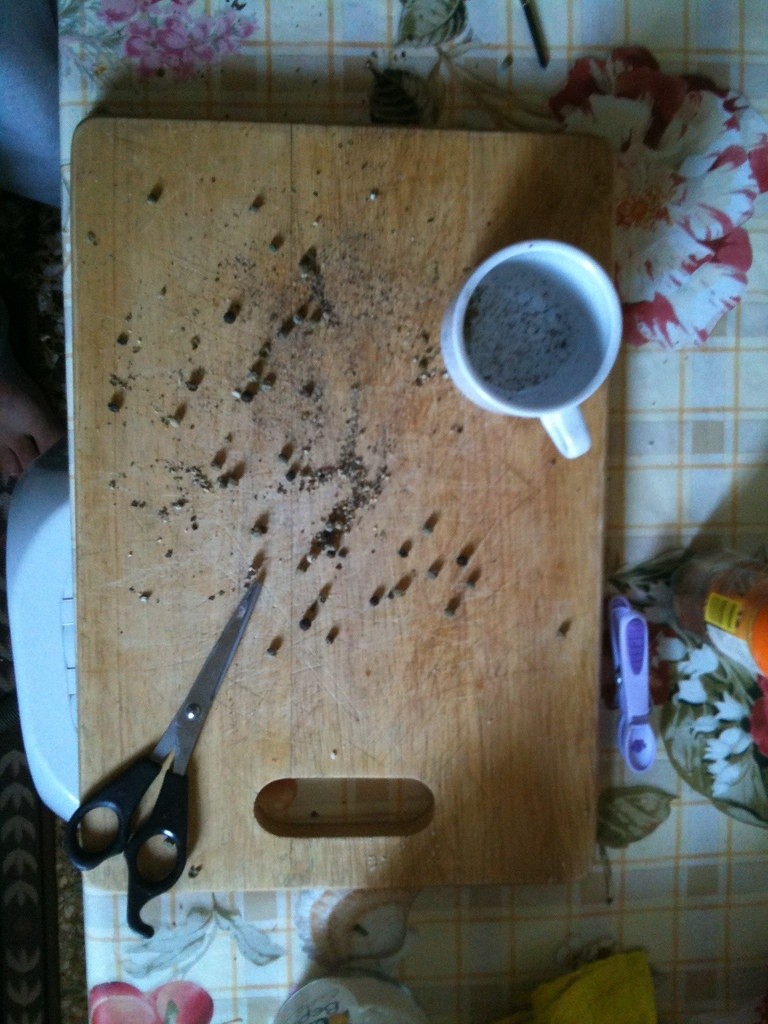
516;0;550;72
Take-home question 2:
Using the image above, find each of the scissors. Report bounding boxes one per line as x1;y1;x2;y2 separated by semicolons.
62;572;264;939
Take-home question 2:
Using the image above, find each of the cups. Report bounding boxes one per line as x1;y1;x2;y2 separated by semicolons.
440;239;624;460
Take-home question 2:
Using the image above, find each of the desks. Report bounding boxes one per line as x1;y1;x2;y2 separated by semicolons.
53;0;768;1024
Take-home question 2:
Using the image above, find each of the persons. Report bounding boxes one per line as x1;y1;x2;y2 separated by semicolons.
0;0;71;480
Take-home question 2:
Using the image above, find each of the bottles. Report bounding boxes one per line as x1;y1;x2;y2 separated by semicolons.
669;546;768;680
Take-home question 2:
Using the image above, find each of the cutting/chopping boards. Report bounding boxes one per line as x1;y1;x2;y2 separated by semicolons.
70;120;610;890
